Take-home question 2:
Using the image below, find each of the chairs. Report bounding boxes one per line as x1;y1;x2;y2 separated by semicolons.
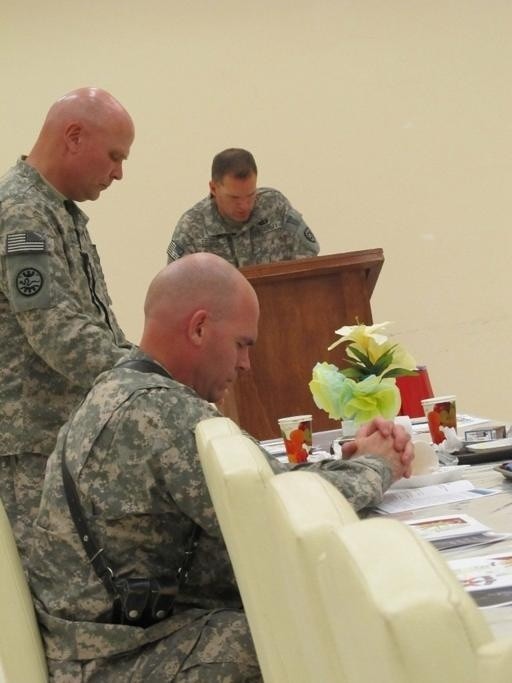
194;417;511;683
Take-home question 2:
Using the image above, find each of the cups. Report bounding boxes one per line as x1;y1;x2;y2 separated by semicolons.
275;412;314;465
419;393;458;445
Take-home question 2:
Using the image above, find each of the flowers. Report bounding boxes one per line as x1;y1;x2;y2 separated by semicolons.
310;316;419;423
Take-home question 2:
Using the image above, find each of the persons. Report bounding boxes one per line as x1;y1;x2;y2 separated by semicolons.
2;86;139;557
24;251;415;683
167;147;320;268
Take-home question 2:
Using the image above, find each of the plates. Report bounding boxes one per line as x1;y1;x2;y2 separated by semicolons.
463;438;512;454
